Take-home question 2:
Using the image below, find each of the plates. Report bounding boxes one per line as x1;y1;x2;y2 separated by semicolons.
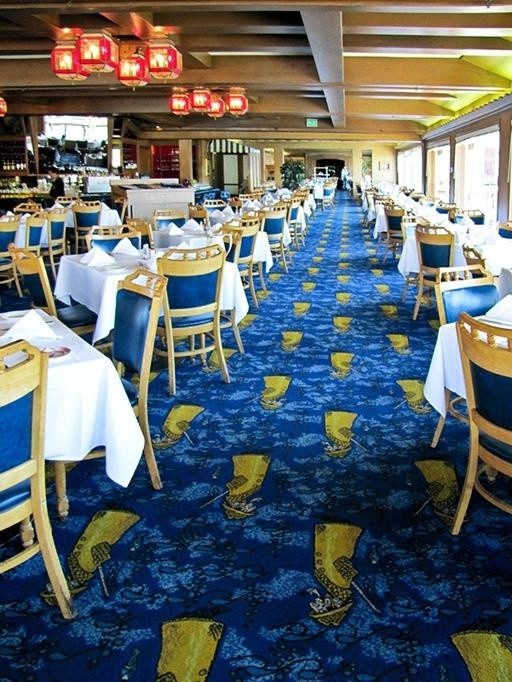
38;346;72;360
1;310;33;320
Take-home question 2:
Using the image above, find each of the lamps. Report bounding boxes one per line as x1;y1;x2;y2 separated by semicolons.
169;85;248;120
0;98;9;117
51;25;183;92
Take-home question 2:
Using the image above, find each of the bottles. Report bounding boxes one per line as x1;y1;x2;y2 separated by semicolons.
2;159;26;171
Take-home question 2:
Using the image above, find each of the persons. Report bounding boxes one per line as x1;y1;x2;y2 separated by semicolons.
48;167;65;205
